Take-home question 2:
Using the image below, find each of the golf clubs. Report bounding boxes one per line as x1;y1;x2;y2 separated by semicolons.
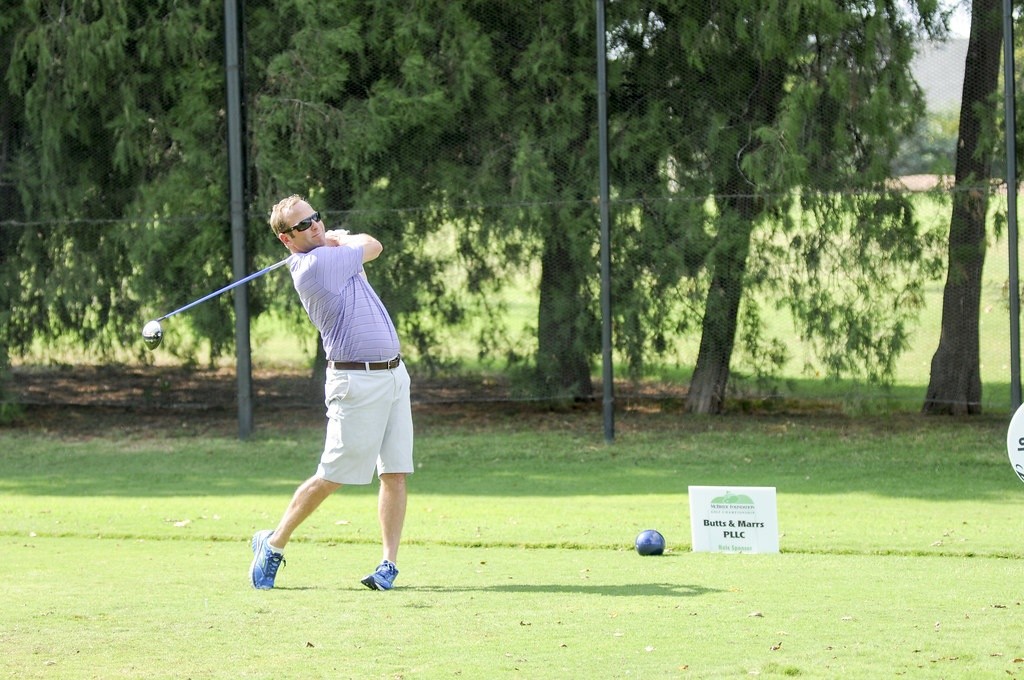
141;259;285;350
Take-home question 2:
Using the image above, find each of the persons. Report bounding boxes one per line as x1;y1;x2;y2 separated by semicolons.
250;194;415;591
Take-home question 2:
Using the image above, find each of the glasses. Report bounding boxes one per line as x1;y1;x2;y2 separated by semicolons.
281;211;321;233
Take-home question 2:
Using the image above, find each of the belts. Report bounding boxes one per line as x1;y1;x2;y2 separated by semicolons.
327;353;401;370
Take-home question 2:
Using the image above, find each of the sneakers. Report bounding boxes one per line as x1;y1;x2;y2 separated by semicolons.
360;560;399;592
250;529;287;590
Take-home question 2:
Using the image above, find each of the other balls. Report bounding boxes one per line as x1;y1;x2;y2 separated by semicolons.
635;530;666;555
